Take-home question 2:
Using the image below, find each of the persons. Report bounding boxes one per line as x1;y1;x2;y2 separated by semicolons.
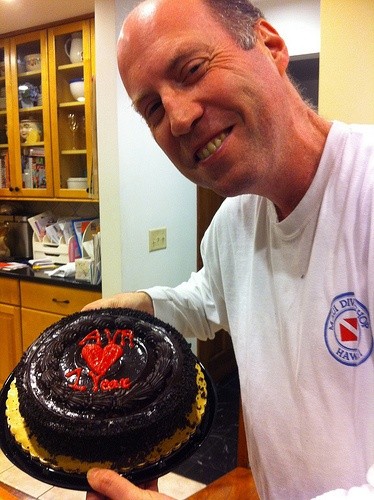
81;0;374;500
20;120;41;143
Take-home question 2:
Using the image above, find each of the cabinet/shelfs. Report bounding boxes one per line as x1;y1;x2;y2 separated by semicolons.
0;276;101;389
1;13;98;202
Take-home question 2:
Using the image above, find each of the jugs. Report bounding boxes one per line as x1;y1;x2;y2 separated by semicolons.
65;32;83;64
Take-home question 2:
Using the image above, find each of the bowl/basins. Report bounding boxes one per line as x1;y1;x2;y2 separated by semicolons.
23;53;40;71
69;77;85;101
66;178;88;189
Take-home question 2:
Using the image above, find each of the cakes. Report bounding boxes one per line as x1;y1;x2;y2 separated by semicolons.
14;306;198;464
